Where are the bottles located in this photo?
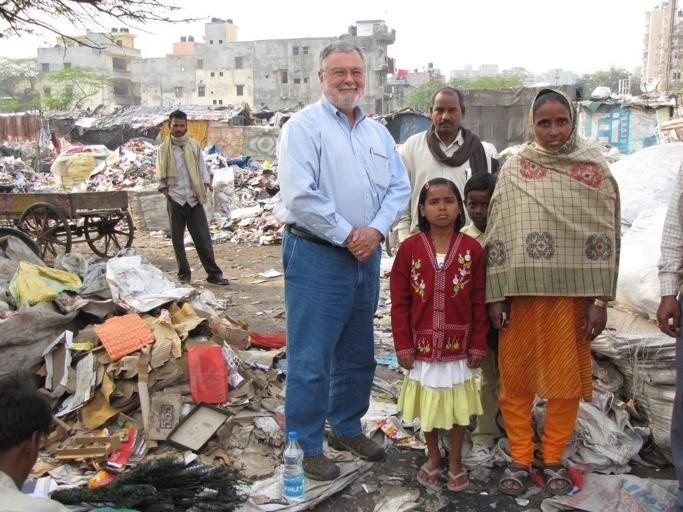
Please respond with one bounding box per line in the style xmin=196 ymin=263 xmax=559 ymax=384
xmin=283 ymin=431 xmax=305 ymax=504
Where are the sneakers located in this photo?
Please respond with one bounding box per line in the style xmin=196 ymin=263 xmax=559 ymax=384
xmin=328 ymin=430 xmax=385 ymax=461
xmin=177 ymin=277 xmax=191 ymax=284
xmin=206 ymin=276 xmax=228 ymax=285
xmin=303 ymin=454 xmax=340 ymax=481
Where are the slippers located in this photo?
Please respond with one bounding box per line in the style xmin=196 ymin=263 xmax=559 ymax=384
xmin=542 ymin=464 xmax=572 ymax=495
xmin=499 ymin=464 xmax=529 ymax=495
xmin=416 ymin=462 xmax=470 ymax=492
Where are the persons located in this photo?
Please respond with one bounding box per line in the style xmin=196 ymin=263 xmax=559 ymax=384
xmin=157 ymin=110 xmax=229 ymax=284
xmin=656 ymin=155 xmax=683 ymax=512
xmin=0 ymin=369 xmax=73 ymax=512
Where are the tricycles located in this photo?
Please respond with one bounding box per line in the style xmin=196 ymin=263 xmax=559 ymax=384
xmin=0 ymin=188 xmax=136 ymax=268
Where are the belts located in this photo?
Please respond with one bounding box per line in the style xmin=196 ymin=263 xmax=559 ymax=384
xmin=290 ymin=224 xmax=339 ymax=248
